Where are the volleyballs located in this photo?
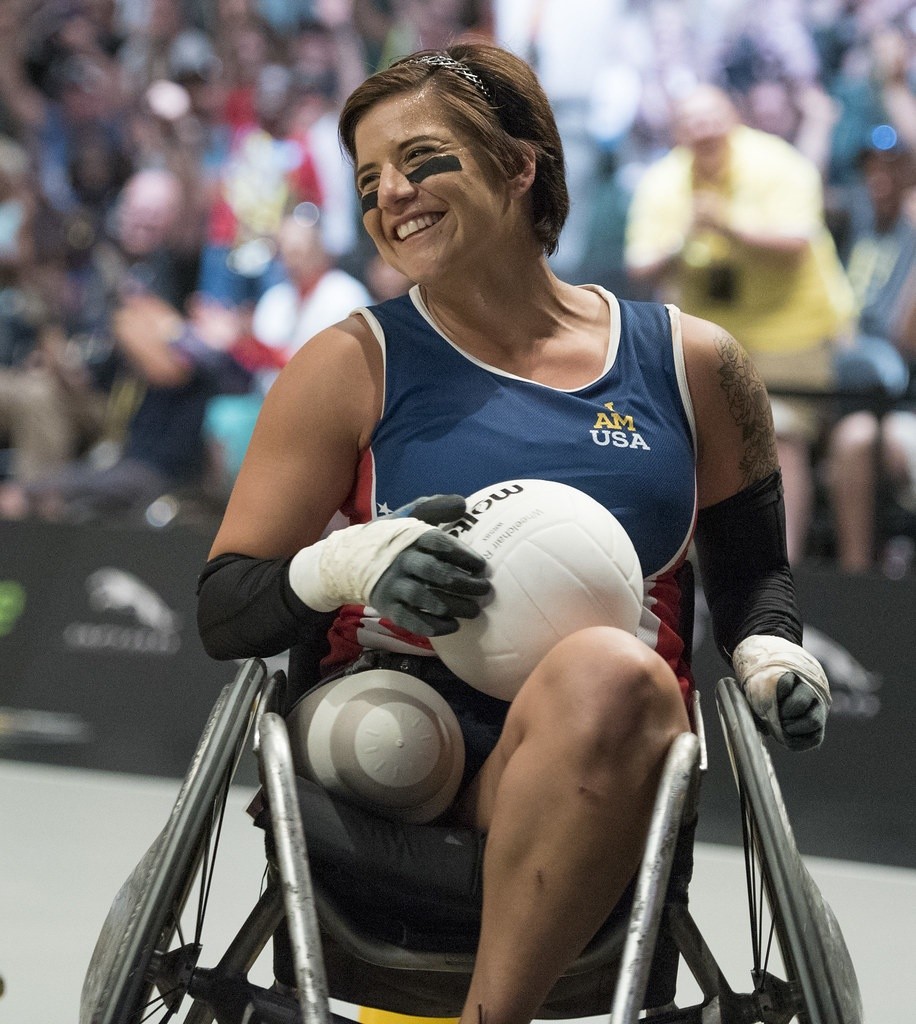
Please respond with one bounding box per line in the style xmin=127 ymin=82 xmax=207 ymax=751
xmin=415 ymin=474 xmax=651 ymax=705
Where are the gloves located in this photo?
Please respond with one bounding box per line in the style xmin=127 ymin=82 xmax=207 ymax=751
xmin=289 ymin=494 xmax=490 ymax=638
xmin=734 ymin=634 xmax=829 ymax=753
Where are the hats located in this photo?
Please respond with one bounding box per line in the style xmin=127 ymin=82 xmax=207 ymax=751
xmin=671 ymin=87 xmax=735 ymax=145
xmin=168 ymin=30 xmax=217 ymax=81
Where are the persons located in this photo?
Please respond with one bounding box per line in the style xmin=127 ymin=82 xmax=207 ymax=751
xmin=196 ymin=45 xmax=827 ymax=1024
xmin=622 ymin=1 xmax=916 ymax=570
xmin=0 ymin=1 xmax=502 ymax=519
